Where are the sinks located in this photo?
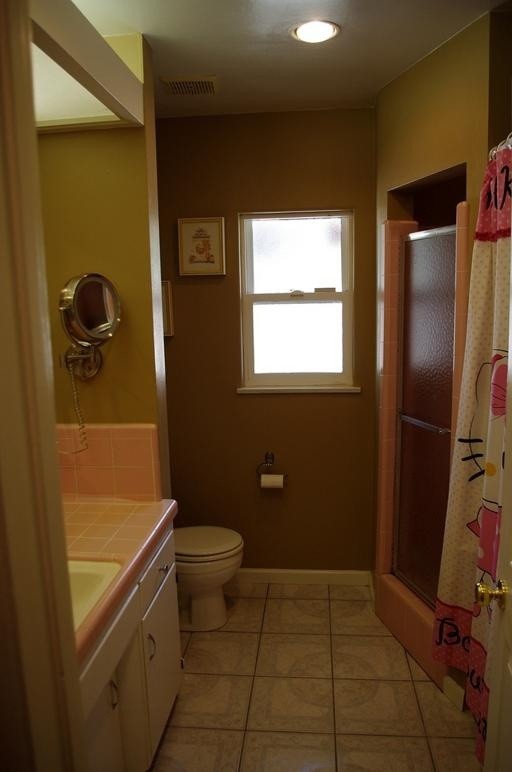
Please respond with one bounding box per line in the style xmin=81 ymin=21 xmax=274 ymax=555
xmin=67 ymin=559 xmax=122 ymax=633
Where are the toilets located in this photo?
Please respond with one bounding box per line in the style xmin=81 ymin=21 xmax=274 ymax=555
xmin=172 ymin=525 xmax=246 ymax=633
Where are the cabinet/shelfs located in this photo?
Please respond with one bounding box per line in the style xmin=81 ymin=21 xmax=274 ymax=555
xmin=72 ymin=516 xmax=185 ymax=772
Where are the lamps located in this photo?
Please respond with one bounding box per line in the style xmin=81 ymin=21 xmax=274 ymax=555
xmin=290 ymin=21 xmax=340 ymax=44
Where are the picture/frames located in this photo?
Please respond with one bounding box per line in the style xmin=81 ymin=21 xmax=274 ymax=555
xmin=177 ymin=217 xmax=226 ymax=277
xmin=161 ymin=280 xmax=175 ymax=337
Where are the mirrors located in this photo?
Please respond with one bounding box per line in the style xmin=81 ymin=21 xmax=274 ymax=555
xmin=58 ymin=273 xmax=124 ymax=381
